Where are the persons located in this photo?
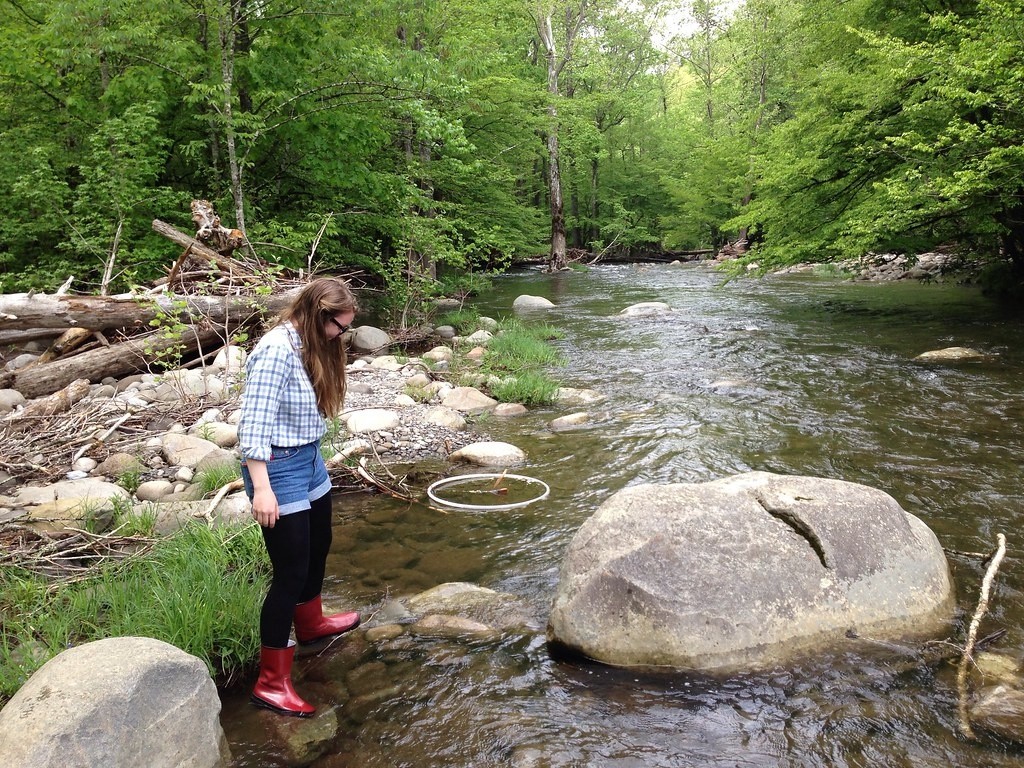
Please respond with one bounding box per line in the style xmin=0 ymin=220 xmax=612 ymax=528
xmin=237 ymin=277 xmax=361 ymax=714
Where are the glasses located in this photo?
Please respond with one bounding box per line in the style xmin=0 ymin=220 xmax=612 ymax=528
xmin=330 ymin=316 xmax=349 ymax=335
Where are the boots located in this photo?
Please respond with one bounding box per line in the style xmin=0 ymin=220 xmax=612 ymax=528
xmin=249 ymin=639 xmax=316 ymax=716
xmin=292 ymin=593 xmax=361 ymax=646
